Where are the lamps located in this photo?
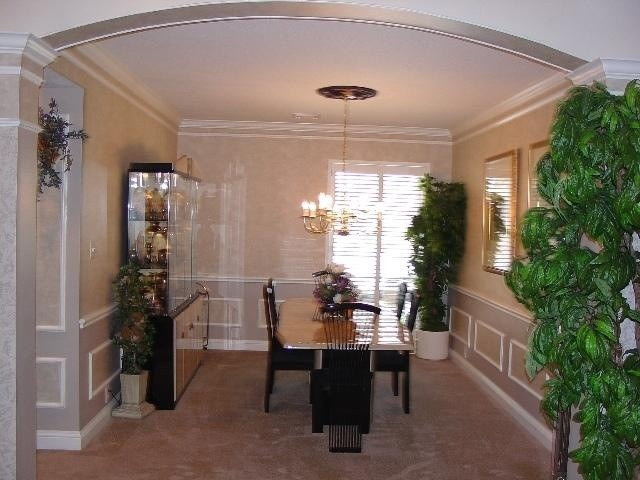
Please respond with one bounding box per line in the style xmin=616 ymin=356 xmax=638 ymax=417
xmin=301 ymin=85 xmax=385 ymax=236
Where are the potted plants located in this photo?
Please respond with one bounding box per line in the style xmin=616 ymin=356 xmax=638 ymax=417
xmin=405 ymin=172 xmax=468 ymax=360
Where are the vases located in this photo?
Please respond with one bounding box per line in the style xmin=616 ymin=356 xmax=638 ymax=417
xmin=113 ymin=370 xmax=155 ymax=418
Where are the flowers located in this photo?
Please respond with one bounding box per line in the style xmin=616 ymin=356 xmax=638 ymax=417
xmin=108 ymin=262 xmax=155 ymax=374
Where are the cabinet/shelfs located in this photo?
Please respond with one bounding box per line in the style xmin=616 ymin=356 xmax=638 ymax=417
xmin=147 ymin=294 xmax=210 ymax=411
xmin=126 ymin=158 xmax=201 ymax=316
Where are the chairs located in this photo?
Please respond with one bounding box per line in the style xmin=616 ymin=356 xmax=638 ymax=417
xmin=262 ymin=270 xmax=421 ymax=434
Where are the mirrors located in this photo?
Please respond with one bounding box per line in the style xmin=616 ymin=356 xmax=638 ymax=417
xmin=481 ymin=148 xmax=519 ymax=276
xmin=527 ymin=139 xmax=552 ymax=208
